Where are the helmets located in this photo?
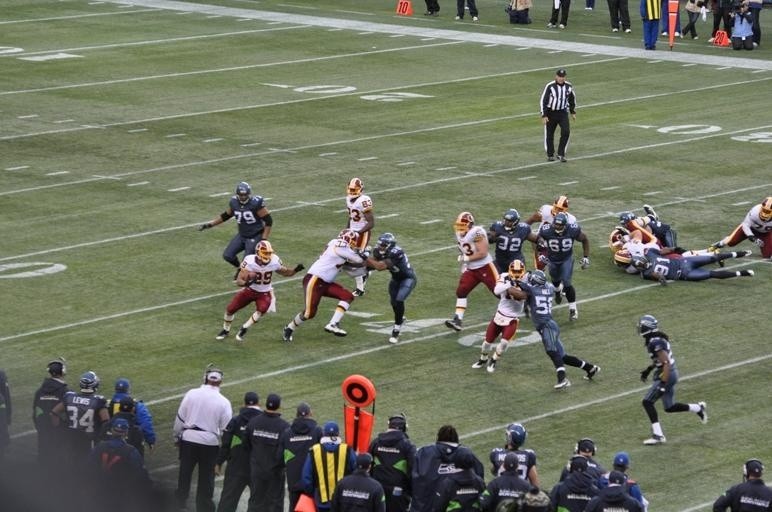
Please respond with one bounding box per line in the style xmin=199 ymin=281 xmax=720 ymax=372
xmin=528 ymin=270 xmax=546 ymax=286
xmin=613 ymin=250 xmax=633 ymax=268
xmin=375 ymin=232 xmax=396 ymax=257
xmin=503 ymin=208 xmax=520 ymax=232
xmin=255 ymin=240 xmax=273 ymax=265
xmin=608 ymin=229 xmax=624 ymax=254
xmin=80 ymin=371 xmax=101 ymax=394
xmin=506 ymin=422 xmax=527 ymax=449
xmin=636 ymin=314 xmax=658 ymax=337
xmin=550 ymin=195 xmax=570 ymax=217
xmin=552 ymin=214 xmax=568 ymax=235
xmin=760 ymin=197 xmax=771 ymax=221
xmin=619 ymin=212 xmax=636 ymax=227
xmin=346 ymin=177 xmax=364 ymax=198
xmin=454 ymin=211 xmax=475 ymax=237
xmin=508 ymin=259 xmax=525 ymax=280
xmin=338 ymin=229 xmax=358 ymax=251
xmin=234 ymin=181 xmax=252 ymax=206
xmin=47 ymin=358 xmax=66 ymax=378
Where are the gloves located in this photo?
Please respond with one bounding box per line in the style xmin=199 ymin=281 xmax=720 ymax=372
xmin=195 ymin=223 xmax=212 ymax=231
xmin=363 ymin=244 xmax=372 ymax=254
xmin=660 ymin=277 xmax=667 ymax=287
xmin=456 ymin=255 xmax=470 ymax=264
xmin=355 ymin=252 xmax=368 ymax=262
xmin=580 ymin=255 xmax=590 ymax=269
xmin=753 ymin=238 xmax=765 ymax=248
xmin=675 ymin=247 xmax=687 ymax=255
xmin=536 ymin=243 xmax=545 ymax=252
xmin=538 ymin=255 xmax=550 ymax=266
xmin=295 ymin=263 xmax=305 ymax=273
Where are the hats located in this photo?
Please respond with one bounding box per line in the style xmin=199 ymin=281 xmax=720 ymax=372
xmin=386 ymin=412 xmax=407 ymax=425
xmin=504 ymin=452 xmax=518 ymax=472
xmin=203 ymin=365 xmax=223 ymax=385
xmin=743 ymin=459 xmax=763 ymax=478
xmin=567 ymin=439 xmax=628 ymax=485
xmin=357 ymin=453 xmax=377 ymax=471
xmin=556 ymin=69 xmax=567 ymax=76
xmin=244 ymin=391 xmax=339 ymax=439
xmin=519 ymin=487 xmax=553 ymax=510
xmin=110 ymin=377 xmax=136 ymax=433
xmin=453 ymin=448 xmax=477 ymax=470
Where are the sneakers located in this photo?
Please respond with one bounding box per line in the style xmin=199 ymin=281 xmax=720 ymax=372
xmin=546 ymin=155 xmax=567 ymax=163
xmin=472 ymin=360 xmax=487 ymax=368
xmin=644 ymin=204 xmax=659 ymax=222
xmin=554 ymin=379 xmax=571 ymax=389
xmin=583 ymin=365 xmax=601 ymax=380
xmin=235 ymin=324 xmax=246 ymax=341
xmin=554 ymin=290 xmax=563 ymax=304
xmin=706 ymin=240 xmax=754 ymax=278
xmin=395 ymin=316 xmax=406 ymax=330
xmin=281 ymin=323 xmax=294 ymax=344
xmin=643 ymin=434 xmax=667 ymax=446
xmin=698 ymin=402 xmax=708 ymax=426
xmin=443 ymin=316 xmax=462 ymax=331
xmin=568 ymin=307 xmax=578 ymax=322
xmin=389 ymin=329 xmax=400 ymax=344
xmin=487 ymin=360 xmax=497 ymax=373
xmin=323 ymin=322 xmax=348 ymax=338
xmin=353 ymin=289 xmax=365 ymax=298
xmin=216 ymin=329 xmax=230 ymax=340
xmin=423 ymin=6 xmax=759 ymax=50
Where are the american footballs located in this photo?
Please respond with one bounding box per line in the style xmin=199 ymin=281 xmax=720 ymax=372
xmin=248 ymin=272 xmax=256 ymax=280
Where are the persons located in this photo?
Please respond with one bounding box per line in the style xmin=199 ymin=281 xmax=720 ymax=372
xmin=200 ymin=179 xmax=417 ymax=344
xmin=445 ymin=195 xmax=600 ymax=390
xmin=423 ymin=0 xmax=764 ymax=50
xmin=712 ymin=458 xmax=771 ymax=512
xmin=609 ymin=177 xmax=771 ymax=282
xmin=539 ymin=69 xmax=577 ymax=162
xmin=636 ymin=314 xmax=707 ymax=445
xmin=1 ymin=360 xmax=649 ymax=511
